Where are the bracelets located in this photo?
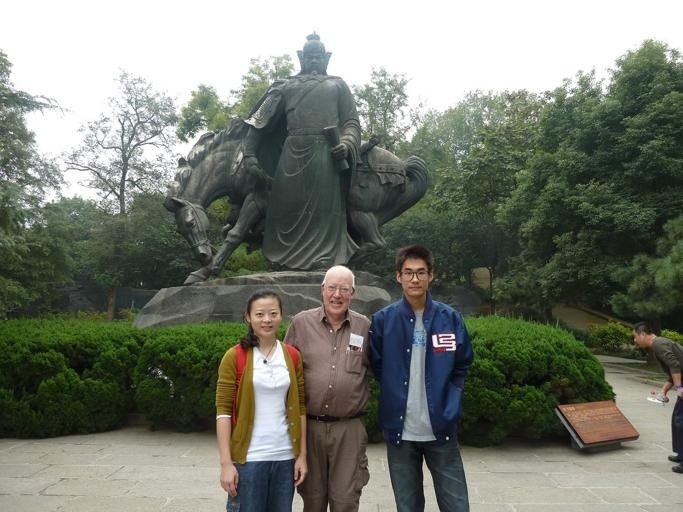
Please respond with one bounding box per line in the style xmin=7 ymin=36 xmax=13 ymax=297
xmin=673 ymin=384 xmax=681 ymax=389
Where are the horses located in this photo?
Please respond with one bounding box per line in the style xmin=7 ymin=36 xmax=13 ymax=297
xmin=157 ymin=113 xmax=431 ymax=286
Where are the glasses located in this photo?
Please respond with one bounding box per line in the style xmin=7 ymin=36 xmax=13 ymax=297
xmin=398 ymin=269 xmax=430 ymax=282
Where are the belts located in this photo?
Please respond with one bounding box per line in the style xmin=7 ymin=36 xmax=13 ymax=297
xmin=302 ymin=410 xmax=370 ymax=424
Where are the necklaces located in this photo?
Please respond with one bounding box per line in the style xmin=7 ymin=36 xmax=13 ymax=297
xmin=263 ymin=340 xmax=276 ymax=364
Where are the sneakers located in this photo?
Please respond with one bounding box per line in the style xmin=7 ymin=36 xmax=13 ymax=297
xmin=668 ymin=453 xmax=683 ymax=473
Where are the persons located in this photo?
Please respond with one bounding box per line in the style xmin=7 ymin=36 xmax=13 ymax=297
xmin=240 ymin=28 xmax=364 ymax=272
xmin=282 ymin=265 xmax=373 ymax=512
xmin=214 ymin=291 xmax=308 ymax=512
xmin=367 ymin=245 xmax=475 ymax=512
xmin=632 ymin=321 xmax=683 ymax=473
